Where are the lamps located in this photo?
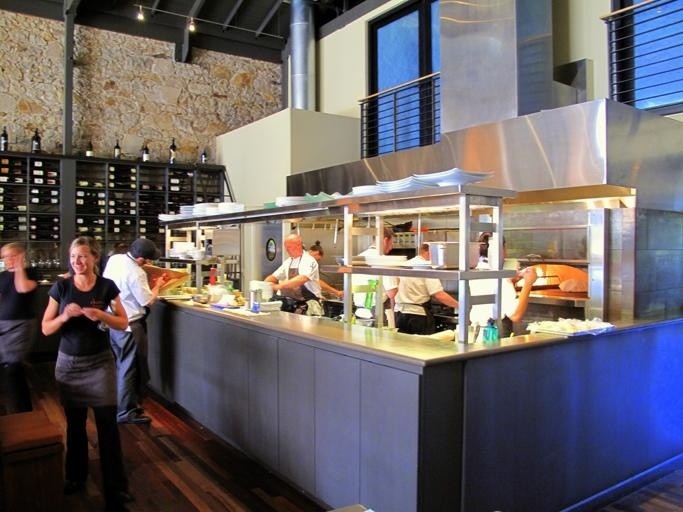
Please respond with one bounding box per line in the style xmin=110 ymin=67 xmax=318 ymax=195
xmin=136 ymin=3 xmax=145 ymax=22
xmin=188 ymin=16 xmax=198 ymax=33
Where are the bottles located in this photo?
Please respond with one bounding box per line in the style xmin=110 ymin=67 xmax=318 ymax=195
xmin=0 ymin=126 xmax=221 ymax=241
xmin=482 ymin=318 xmax=499 ymax=348
xmin=249 ymin=291 xmax=262 ymax=313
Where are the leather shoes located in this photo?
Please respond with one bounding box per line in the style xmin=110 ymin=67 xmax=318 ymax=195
xmin=118 ymin=408 xmax=152 ymax=424
xmin=64 ymin=480 xmax=132 ymax=504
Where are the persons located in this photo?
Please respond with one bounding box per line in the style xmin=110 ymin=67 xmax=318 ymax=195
xmin=466 ymin=233 xmax=537 ymax=337
xmin=264 ymin=234 xmax=343 ymax=315
xmin=101 ymin=239 xmax=166 ymax=423
xmin=41 ymin=236 xmax=135 ymax=503
xmin=349 ymin=229 xmax=397 ymax=327
xmin=0 ymin=242 xmax=47 ymax=413
xmin=395 ymin=242 xmax=458 ymax=334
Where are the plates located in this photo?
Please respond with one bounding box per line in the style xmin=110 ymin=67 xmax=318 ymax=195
xmin=275 ymin=196 xmax=305 ymax=207
xmin=352 ymin=167 xmax=495 ymax=196
xmin=158 ymin=201 xmax=244 ymax=222
xmin=366 ymin=255 xmax=407 ymax=270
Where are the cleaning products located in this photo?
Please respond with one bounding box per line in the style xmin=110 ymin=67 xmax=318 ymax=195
xmin=484 ymin=319 xmax=499 ymax=346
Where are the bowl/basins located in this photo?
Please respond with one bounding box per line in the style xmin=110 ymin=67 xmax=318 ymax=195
xmin=423 ymin=240 xmax=486 ymax=270
xmin=192 ymin=294 xmax=211 ymax=305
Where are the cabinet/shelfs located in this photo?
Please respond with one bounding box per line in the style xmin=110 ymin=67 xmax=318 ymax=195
xmin=0 ymin=151 xmax=225 ymax=282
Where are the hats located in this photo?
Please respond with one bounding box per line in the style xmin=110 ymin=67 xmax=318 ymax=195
xmin=130 ymin=239 xmax=162 ymax=260
